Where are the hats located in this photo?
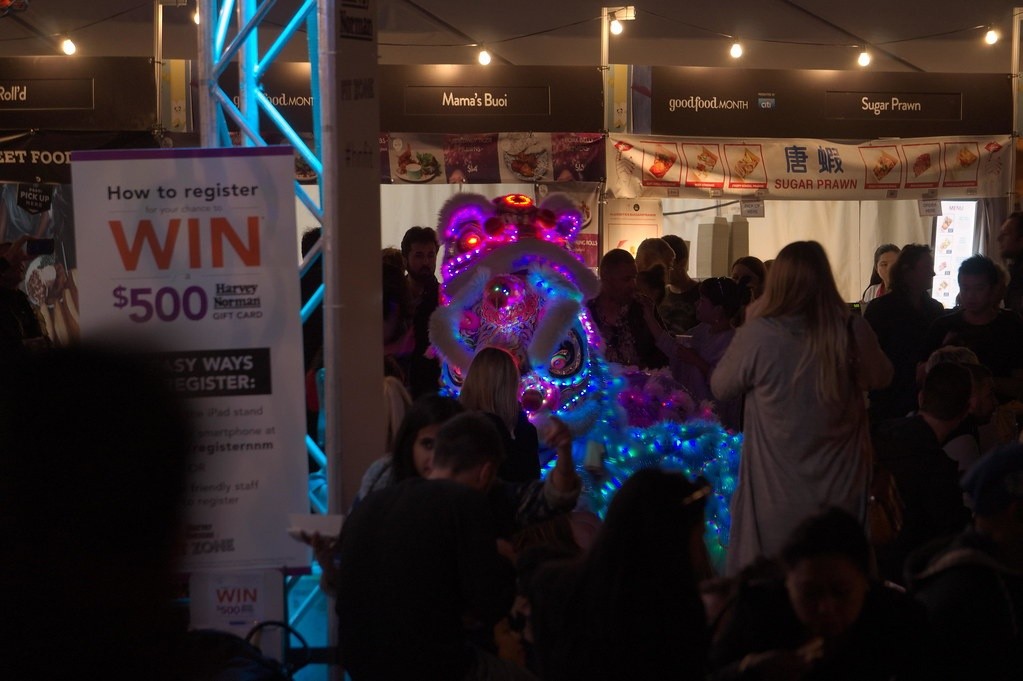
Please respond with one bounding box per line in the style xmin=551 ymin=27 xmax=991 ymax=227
xmin=960 ymin=442 xmax=1023 ymax=516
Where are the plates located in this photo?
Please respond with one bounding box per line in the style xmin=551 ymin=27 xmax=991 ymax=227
xmin=281 ymin=139 xmax=318 ymax=181
xmin=503 ymin=147 xmax=550 ymax=182
xmin=395 ymin=166 xmax=436 ymax=182
xmin=23 ymin=255 xmax=67 ymax=308
xmin=577 ymin=202 xmax=592 ymax=228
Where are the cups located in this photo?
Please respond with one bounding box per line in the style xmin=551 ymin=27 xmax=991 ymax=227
xmin=405 ymin=164 xmax=422 ymax=178
xmin=677 ymin=334 xmax=693 ymax=350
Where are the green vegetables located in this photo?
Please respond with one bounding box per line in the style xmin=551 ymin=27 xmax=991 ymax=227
xmin=417 ymin=153 xmax=442 ymax=175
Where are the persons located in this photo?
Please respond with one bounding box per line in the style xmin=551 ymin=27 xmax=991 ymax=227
xmin=710 ymin=241 xmax=893 ymax=586
xmin=300 ymin=212 xmax=1023 ymax=680
xmin=0 ymin=219 xmax=291 ymax=681
